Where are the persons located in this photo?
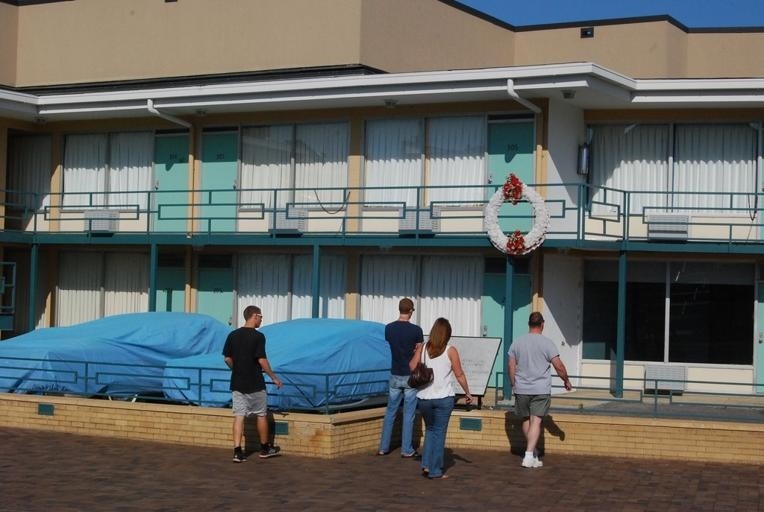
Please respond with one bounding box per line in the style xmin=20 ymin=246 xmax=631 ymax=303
xmin=377 ymin=298 xmax=424 ymax=458
xmin=408 ymin=318 xmax=472 ymax=480
xmin=222 ymin=306 xmax=281 ymax=462
xmin=508 ymin=313 xmax=571 ymax=469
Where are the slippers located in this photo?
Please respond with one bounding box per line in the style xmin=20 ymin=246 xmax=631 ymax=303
xmin=402 ymin=451 xmax=422 ymax=458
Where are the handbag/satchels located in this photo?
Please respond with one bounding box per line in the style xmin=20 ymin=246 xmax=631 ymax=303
xmin=408 ymin=362 xmax=433 ymax=388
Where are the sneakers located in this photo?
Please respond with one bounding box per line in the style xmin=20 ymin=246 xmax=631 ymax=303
xmin=233 ymin=451 xmax=248 ymax=462
xmin=522 ymin=448 xmax=543 ymax=468
xmin=259 ymin=443 xmax=280 ymax=458
